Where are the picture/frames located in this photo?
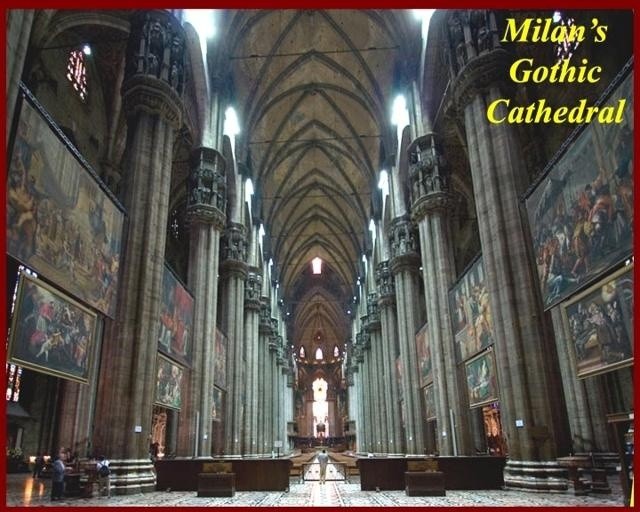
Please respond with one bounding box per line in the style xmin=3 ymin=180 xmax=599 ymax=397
xmin=154 ymin=352 xmax=189 ymax=413
xmin=465 ymin=350 xmax=499 ymax=409
xmin=6 ymin=269 xmax=99 ymax=385
xmin=559 ymin=261 xmax=634 ymax=381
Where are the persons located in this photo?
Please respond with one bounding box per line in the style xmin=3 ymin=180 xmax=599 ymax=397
xmin=317 ymin=449 xmax=329 ymax=485
xmin=95 ymin=455 xmax=111 ymax=499
xmin=567 ymin=434 xmax=600 ymax=484
xmin=31 ymin=443 xmax=75 ymax=501
xmin=415 ymin=123 xmax=633 ymax=455
xmin=6 ymin=128 xmax=228 ymax=420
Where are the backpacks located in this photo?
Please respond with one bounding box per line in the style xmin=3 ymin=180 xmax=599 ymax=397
xmin=99 ymin=460 xmax=111 ymax=478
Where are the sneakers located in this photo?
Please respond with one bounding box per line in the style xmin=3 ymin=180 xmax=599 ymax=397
xmin=50 ymin=497 xmax=64 ymax=502
